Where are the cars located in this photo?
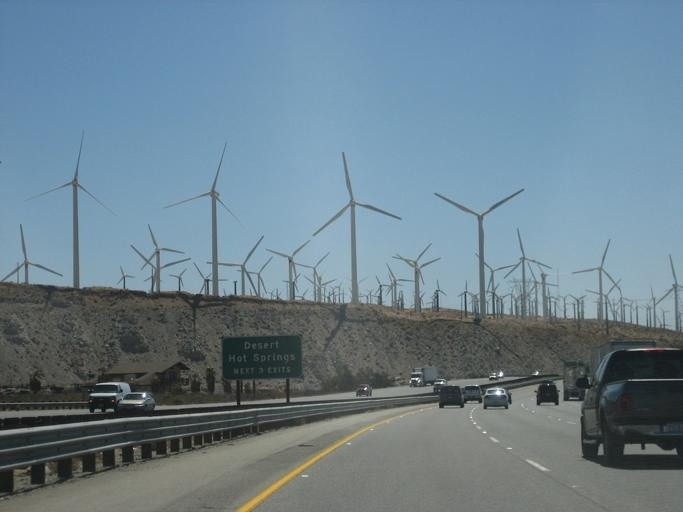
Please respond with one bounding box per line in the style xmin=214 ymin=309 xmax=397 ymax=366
xmin=355 ymin=383 xmax=373 ymax=397
xmin=430 ymin=369 xmax=563 ymax=410
xmin=115 ymin=390 xmax=157 ymax=414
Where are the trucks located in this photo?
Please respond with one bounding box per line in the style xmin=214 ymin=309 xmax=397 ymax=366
xmin=595 ymin=339 xmax=659 ymax=363
xmin=561 ymin=359 xmax=587 ymax=404
xmin=86 ymin=380 xmax=132 ymax=416
xmin=408 ymin=365 xmax=438 ymax=388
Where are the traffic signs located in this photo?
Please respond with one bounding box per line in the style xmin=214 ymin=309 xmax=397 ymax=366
xmin=220 ymin=335 xmax=304 ymax=381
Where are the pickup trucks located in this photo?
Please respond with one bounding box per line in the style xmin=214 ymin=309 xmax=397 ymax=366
xmin=569 ymin=344 xmax=682 ymax=465
